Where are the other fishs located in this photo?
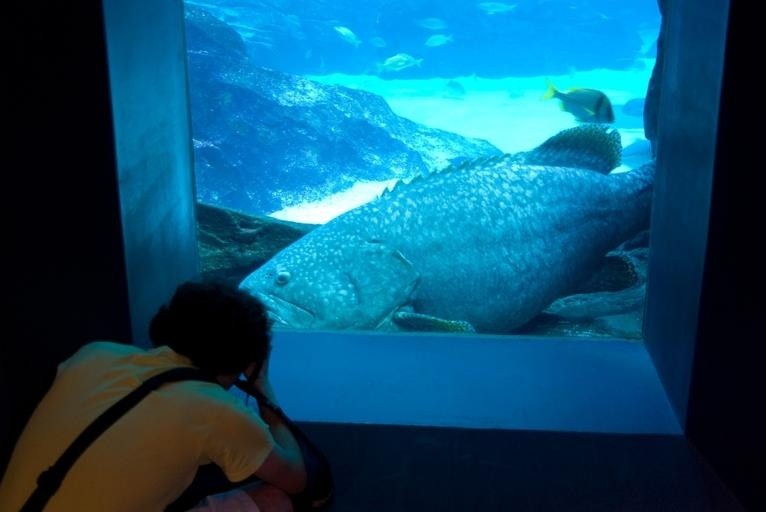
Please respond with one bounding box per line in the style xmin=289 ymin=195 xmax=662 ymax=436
xmin=238 ymin=125 xmax=656 ymax=334
xmin=329 ymin=1 xmax=654 ymax=123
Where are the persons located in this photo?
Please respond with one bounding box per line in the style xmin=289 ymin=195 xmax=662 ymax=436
xmin=0 ymin=279 xmax=308 ymax=511
